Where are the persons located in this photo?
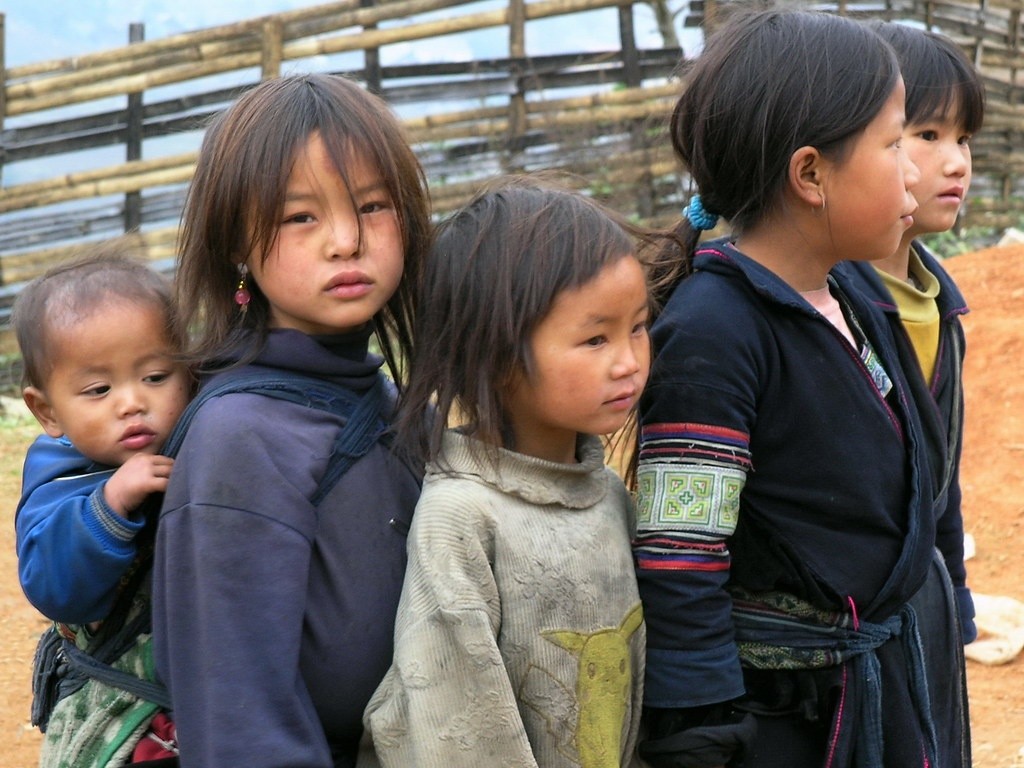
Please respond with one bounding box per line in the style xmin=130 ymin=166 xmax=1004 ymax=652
xmin=13 ymin=247 xmax=191 ymax=768
xmin=152 ymin=70 xmax=440 ymax=768
xmin=354 ymin=187 xmax=648 ymax=767
xmin=857 ymin=13 xmax=987 ymax=768
xmin=608 ymin=7 xmax=974 ymax=768
